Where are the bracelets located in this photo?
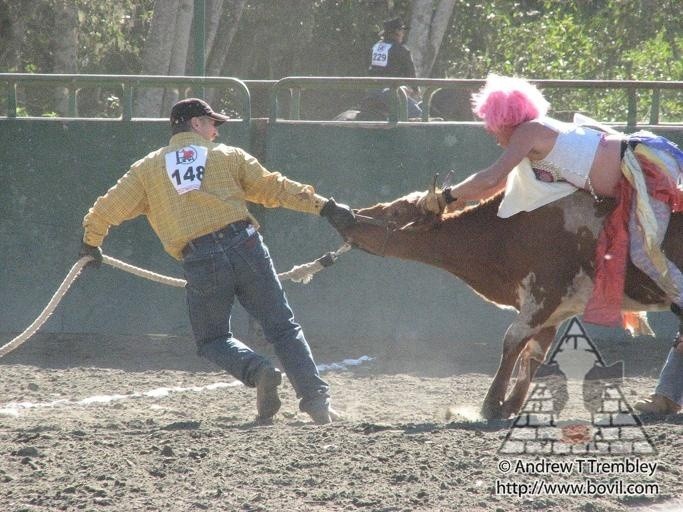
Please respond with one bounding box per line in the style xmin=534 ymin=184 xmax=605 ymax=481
xmin=439 ymin=187 xmax=458 ymax=204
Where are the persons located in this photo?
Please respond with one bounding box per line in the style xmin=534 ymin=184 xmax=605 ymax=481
xmin=361 ymin=16 xmax=423 ymax=121
xmin=631 ymin=332 xmax=681 ymax=417
xmin=413 ymin=72 xmax=682 ymax=357
xmin=77 ymin=97 xmax=362 ymax=426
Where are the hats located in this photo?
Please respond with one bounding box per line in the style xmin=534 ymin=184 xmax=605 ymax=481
xmin=170 ymin=97 xmax=230 ymax=126
xmin=384 ymin=15 xmax=407 ymax=31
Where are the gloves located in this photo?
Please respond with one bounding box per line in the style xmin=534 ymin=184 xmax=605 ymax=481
xmin=320 ymin=197 xmax=357 ymax=226
xmin=79 ymin=244 xmax=102 ymax=268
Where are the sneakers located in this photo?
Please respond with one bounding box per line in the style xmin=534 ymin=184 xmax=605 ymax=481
xmin=309 ymin=406 xmax=331 ymax=423
xmin=634 ymin=394 xmax=681 ymax=415
xmin=256 ymin=367 xmax=282 ymax=417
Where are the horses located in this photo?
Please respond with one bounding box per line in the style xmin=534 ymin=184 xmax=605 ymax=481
xmin=329 ymin=87 xmax=478 ymax=121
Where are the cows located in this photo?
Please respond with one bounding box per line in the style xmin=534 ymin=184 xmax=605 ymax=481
xmin=344 ymin=170 xmax=683 ymax=421
